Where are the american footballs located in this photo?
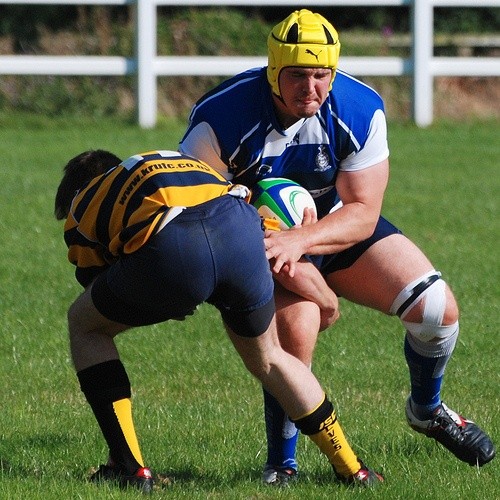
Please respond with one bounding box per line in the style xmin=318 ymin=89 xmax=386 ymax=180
xmin=252 ymin=178 xmax=317 ymax=231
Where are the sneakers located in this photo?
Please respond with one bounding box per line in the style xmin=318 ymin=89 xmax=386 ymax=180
xmin=90 ymin=465 xmax=153 ymax=494
xmin=263 ymin=463 xmax=297 ymax=487
xmin=405 ymin=396 xmax=494 ymax=466
xmin=332 ymin=458 xmax=384 ymax=489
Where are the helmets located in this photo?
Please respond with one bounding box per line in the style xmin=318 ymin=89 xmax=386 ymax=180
xmin=267 ymin=9 xmax=340 ymax=98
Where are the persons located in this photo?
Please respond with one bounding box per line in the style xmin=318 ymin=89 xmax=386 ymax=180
xmin=179 ymin=9 xmax=496 ymax=488
xmin=54 ymin=149 xmax=386 ymax=492
